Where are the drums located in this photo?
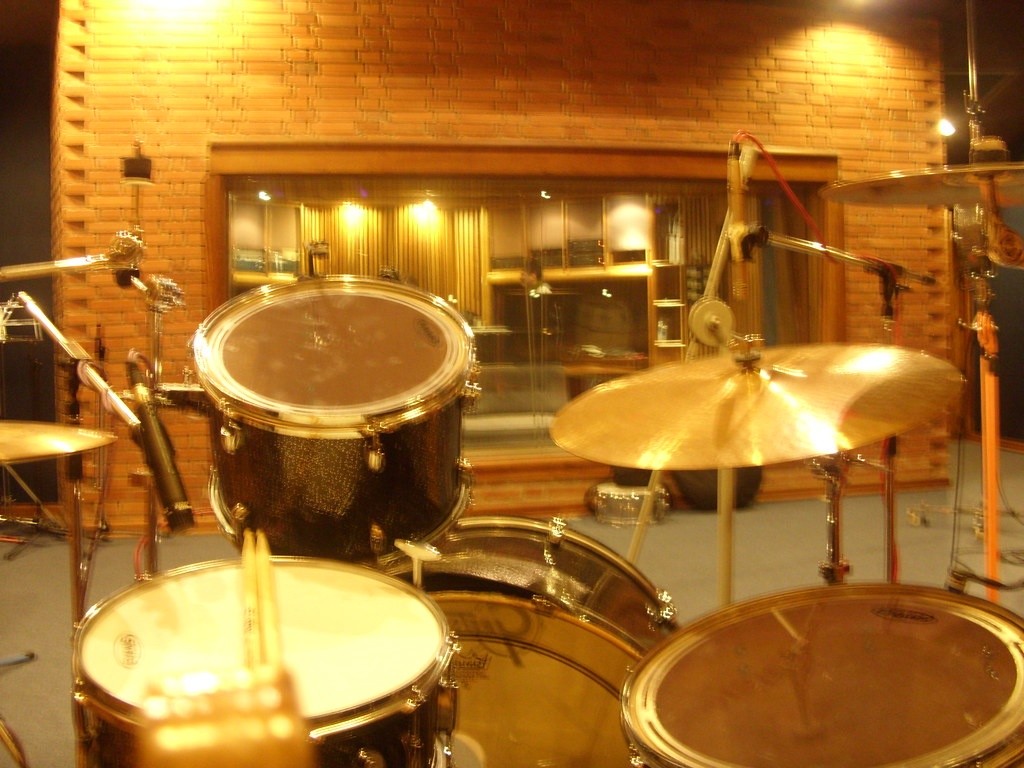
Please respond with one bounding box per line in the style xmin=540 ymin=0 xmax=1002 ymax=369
xmin=366 ymin=513 xmax=679 ymax=768
xmin=69 ymin=554 xmax=461 ymax=768
xmin=593 ymin=482 xmax=669 ymax=525
xmin=616 ymin=582 xmax=1024 ymax=768
xmin=184 ymin=273 xmax=477 ymax=563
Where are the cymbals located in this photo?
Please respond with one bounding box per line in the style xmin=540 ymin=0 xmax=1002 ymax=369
xmin=0 ymin=418 xmax=118 ymax=467
xmin=820 ymin=161 xmax=1024 ymax=210
xmin=547 ymin=342 xmax=966 ymax=473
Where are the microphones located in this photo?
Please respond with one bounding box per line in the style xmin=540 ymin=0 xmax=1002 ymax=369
xmin=727 ymin=141 xmax=756 ymax=266
xmin=123 ymin=361 xmax=196 ymax=536
